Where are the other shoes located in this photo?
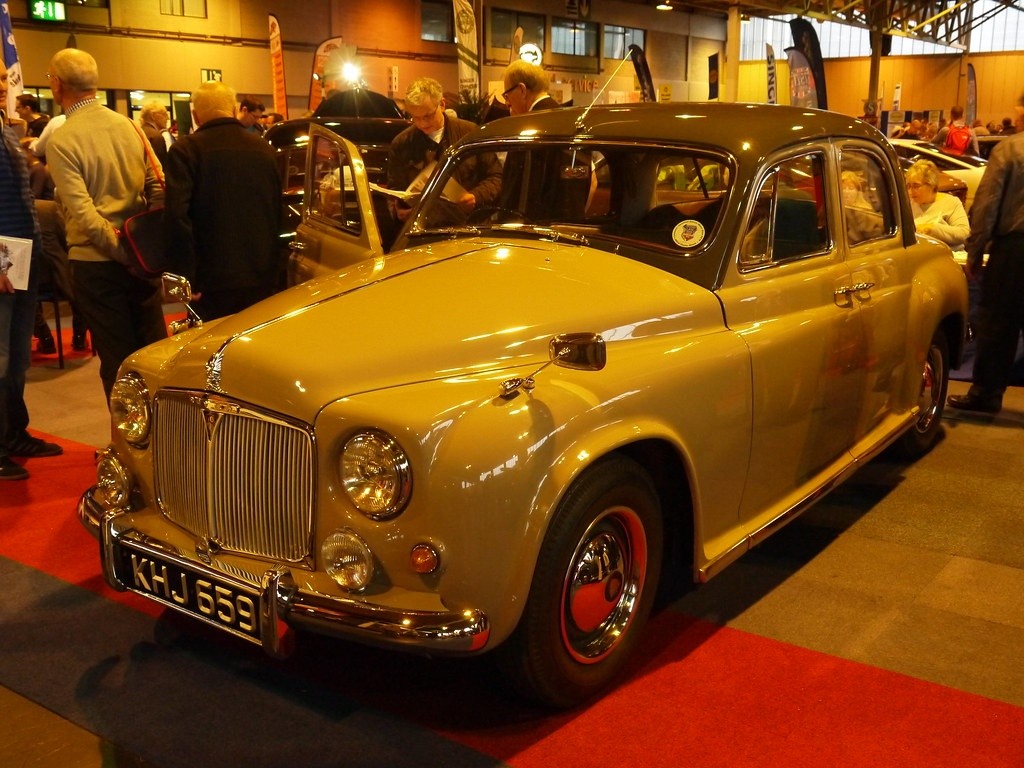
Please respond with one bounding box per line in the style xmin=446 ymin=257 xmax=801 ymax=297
xmin=36 ymin=335 xmax=54 ymax=354
xmin=72 ymin=334 xmax=86 ymax=349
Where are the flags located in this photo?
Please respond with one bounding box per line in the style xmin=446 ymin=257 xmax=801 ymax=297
xmin=0 ymin=0 xmax=25 ymax=120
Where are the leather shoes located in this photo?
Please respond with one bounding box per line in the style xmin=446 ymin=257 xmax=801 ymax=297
xmin=947 ymin=395 xmax=1000 ymax=413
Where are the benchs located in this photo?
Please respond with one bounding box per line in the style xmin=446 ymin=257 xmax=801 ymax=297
xmin=746 ymin=235 xmax=823 ymax=261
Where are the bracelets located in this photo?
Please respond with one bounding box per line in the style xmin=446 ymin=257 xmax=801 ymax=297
xmin=28 ymin=162 xmax=41 ymax=166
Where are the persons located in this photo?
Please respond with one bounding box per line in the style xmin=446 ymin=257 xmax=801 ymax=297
xmin=15 ymin=93 xmax=55 ymax=201
xmin=386 ymin=75 xmax=504 ymax=225
xmin=44 ymin=48 xmax=169 ymax=461
xmin=139 ymin=101 xmax=168 ymax=162
xmin=497 ymin=57 xmax=592 ymax=224
xmin=0 ymin=57 xmax=63 ymax=480
xmin=237 ymin=97 xmax=266 ymax=128
xmin=266 ymin=113 xmax=284 ymax=131
xmin=18 ymin=115 xmax=66 ymax=157
xmin=841 ymin=171 xmax=873 ymax=210
xmin=890 ymin=92 xmax=1024 ymax=411
xmin=33 ymin=200 xmax=90 ymax=353
xmin=166 ymin=80 xmax=284 ymax=329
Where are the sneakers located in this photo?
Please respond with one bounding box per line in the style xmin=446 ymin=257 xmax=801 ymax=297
xmin=0 ymin=457 xmax=28 ymax=478
xmin=7 ymin=434 xmax=62 ymax=456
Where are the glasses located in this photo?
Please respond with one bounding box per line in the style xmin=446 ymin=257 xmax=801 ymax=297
xmin=407 ymin=102 xmax=440 ymax=123
xmin=502 ymin=82 xmax=528 ymax=102
xmin=45 ymin=72 xmax=62 ymax=81
xmin=905 ymin=179 xmax=929 ymax=190
xmin=247 ymin=109 xmax=260 ymax=120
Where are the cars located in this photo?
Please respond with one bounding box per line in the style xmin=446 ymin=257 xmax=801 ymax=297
xmin=260 ymin=113 xmax=407 ymax=225
xmin=585 ymin=135 xmax=989 ymax=223
xmin=75 ymin=103 xmax=970 ymax=717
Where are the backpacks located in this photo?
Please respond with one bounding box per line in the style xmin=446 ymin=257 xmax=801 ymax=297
xmin=946 ymin=123 xmax=972 ymax=153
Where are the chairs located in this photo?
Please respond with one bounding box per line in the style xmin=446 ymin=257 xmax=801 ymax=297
xmin=36 ymin=257 xmax=98 ymax=370
